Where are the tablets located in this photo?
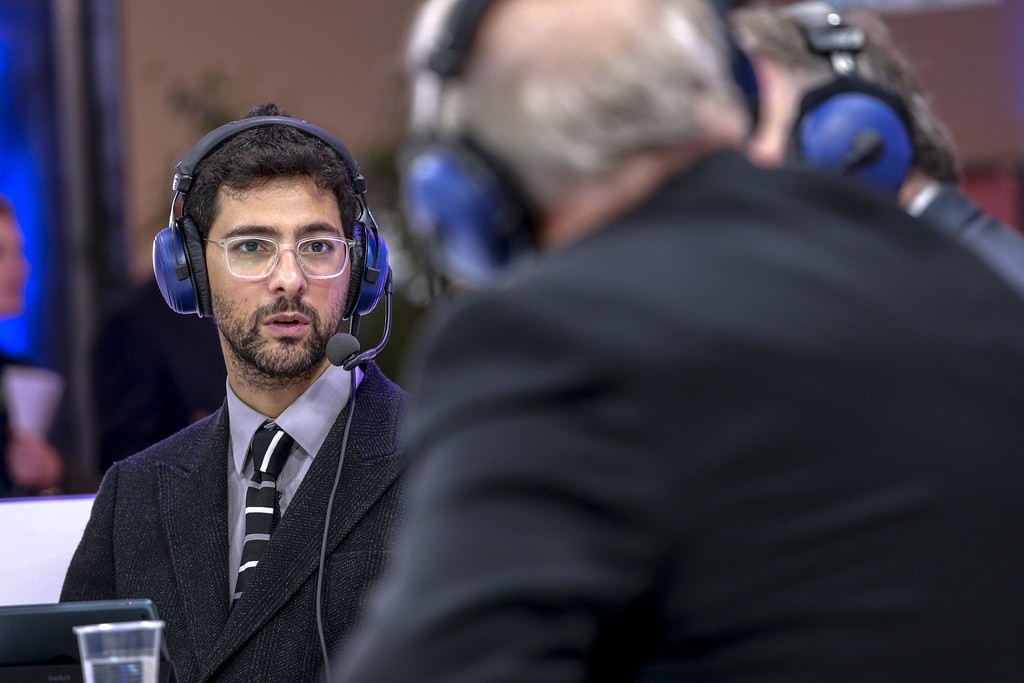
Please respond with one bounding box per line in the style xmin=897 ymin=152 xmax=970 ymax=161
xmin=0 ymin=600 xmax=180 ymax=683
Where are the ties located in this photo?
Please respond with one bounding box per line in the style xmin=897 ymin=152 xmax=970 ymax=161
xmin=230 ymin=424 xmax=299 ymax=617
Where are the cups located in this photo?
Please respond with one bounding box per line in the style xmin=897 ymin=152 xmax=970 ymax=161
xmin=72 ymin=619 xmax=166 ymax=682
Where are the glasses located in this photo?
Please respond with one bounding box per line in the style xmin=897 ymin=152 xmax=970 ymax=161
xmin=203 ymin=234 xmax=357 ymax=282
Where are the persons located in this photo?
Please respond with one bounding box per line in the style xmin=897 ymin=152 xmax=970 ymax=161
xmin=326 ymin=1 xmax=1024 ymax=683
xmin=0 ymin=196 xmax=78 ymax=500
xmin=58 ymin=104 xmax=422 ymax=683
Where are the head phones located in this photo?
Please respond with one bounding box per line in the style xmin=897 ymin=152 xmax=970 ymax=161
xmin=151 ymin=116 xmax=393 ymax=320
xmin=783 ymin=3 xmax=920 ymax=202
xmin=394 ymin=0 xmax=763 ymax=286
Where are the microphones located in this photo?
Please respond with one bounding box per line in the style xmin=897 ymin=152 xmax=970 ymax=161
xmin=326 ymin=293 xmax=393 ymax=371
